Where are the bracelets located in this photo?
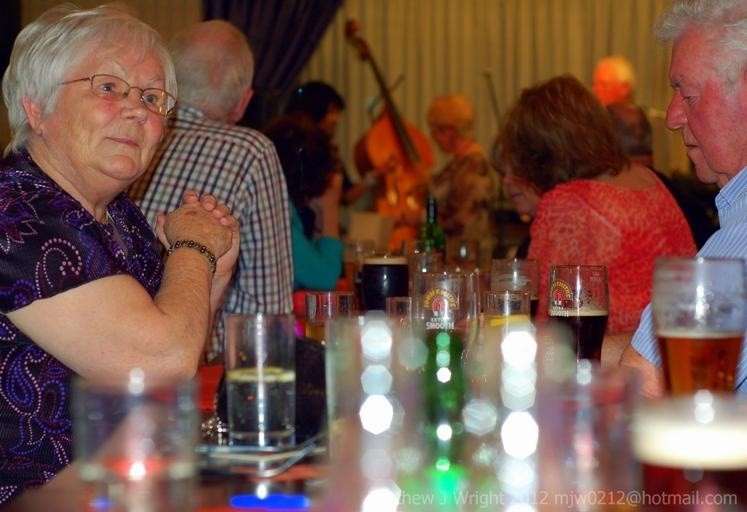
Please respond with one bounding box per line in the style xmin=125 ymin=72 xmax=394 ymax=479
xmin=161 ymin=238 xmax=217 ymax=274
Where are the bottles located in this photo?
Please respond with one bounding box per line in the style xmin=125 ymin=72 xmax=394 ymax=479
xmin=416 ymin=195 xmax=448 ymax=267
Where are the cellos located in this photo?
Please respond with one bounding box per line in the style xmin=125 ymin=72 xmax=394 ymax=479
xmin=340 ymin=18 xmax=437 ymax=250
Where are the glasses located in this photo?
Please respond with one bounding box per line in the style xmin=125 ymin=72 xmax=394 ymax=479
xmin=58 ymin=74 xmax=178 ymax=118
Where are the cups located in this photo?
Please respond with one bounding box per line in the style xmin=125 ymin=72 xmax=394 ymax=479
xmin=67 ymin=232 xmax=747 ymax=511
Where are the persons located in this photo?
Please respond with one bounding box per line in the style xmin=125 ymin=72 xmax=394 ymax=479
xmin=407 ymin=89 xmax=502 ymax=268
xmin=595 ymin=0 xmax=747 ymax=405
xmin=491 ymin=72 xmax=701 ymax=376
xmin=252 ymin=110 xmax=346 ymax=296
xmin=282 ymin=78 xmax=400 ymax=239
xmin=485 ymin=150 xmax=539 ymax=316
xmin=122 ymin=17 xmax=327 ymax=452
xmin=606 ymin=97 xmax=716 ymax=252
xmin=590 ymin=52 xmax=640 ymax=110
xmin=0 ymin=0 xmax=243 ymax=511
